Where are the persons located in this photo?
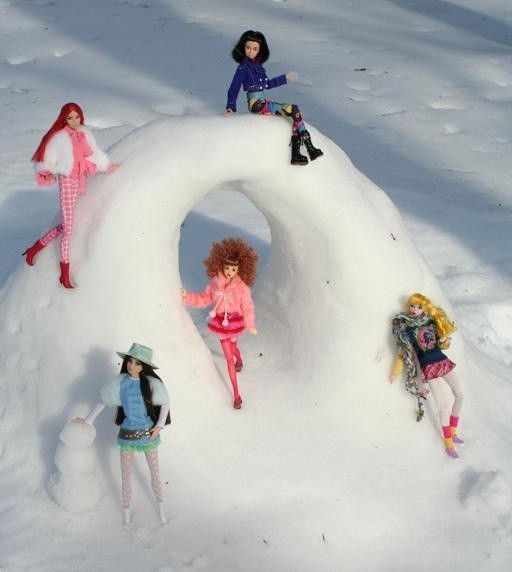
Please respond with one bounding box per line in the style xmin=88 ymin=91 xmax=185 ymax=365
xmin=21 ymin=103 xmax=121 ymax=288
xmin=389 ymin=294 xmax=466 ymax=460
xmin=225 ymin=27 xmax=322 ymax=165
xmin=180 ymin=237 xmax=258 ymax=410
xmin=73 ymin=343 xmax=172 ymax=528
